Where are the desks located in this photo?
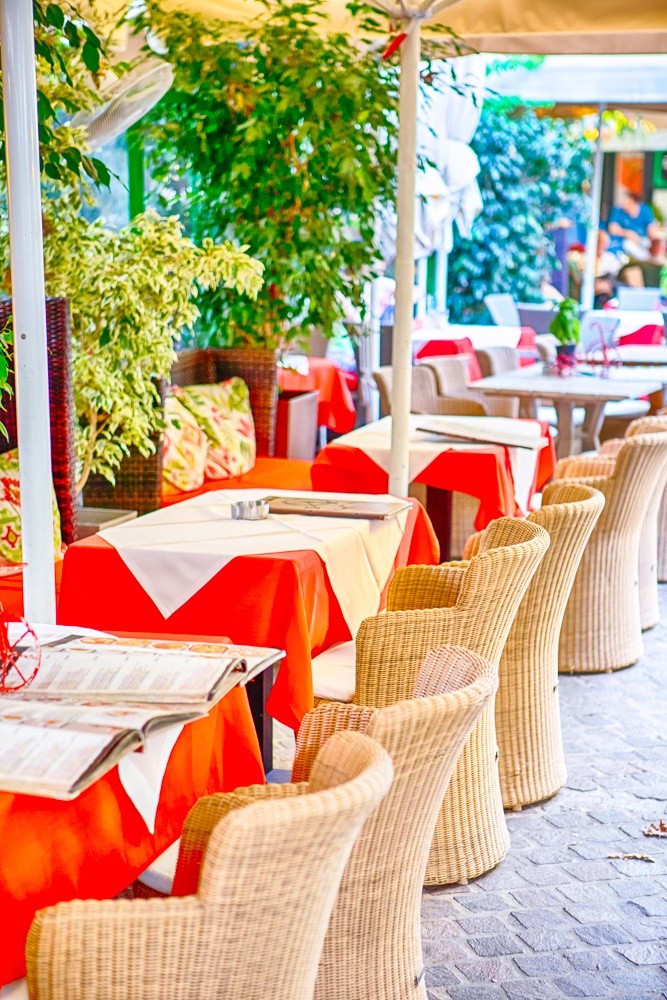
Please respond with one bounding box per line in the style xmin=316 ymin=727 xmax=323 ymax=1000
xmin=63 ymin=487 xmax=442 ymax=774
xmin=467 ymin=345 xmax=665 ymax=453
xmin=0 ymin=630 xmax=265 ymax=986
xmin=311 ymin=412 xmax=557 ymax=561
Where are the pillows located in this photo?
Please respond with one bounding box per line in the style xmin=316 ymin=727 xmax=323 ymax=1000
xmin=181 ymin=378 xmax=255 ymax=480
xmin=161 ymin=394 xmax=206 ymax=494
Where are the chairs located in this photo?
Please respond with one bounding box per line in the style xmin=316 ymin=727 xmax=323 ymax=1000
xmin=24 ymin=286 xmax=667 ymax=1000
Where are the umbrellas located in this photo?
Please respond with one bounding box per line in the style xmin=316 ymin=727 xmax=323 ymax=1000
xmin=0 ymin=0 xmax=667 ymax=625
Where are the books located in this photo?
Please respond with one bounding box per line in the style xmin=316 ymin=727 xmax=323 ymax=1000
xmin=258 ymin=496 xmax=413 ymax=520
xmin=415 ymin=419 xmax=548 ymax=451
xmin=0 ymin=632 xmax=287 ymax=801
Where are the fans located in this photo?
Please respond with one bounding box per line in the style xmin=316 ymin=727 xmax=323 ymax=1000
xmin=70 ymin=59 xmax=178 ymax=151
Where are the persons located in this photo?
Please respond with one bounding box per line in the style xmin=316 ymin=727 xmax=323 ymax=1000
xmin=571 ymin=190 xmax=667 ymax=300
